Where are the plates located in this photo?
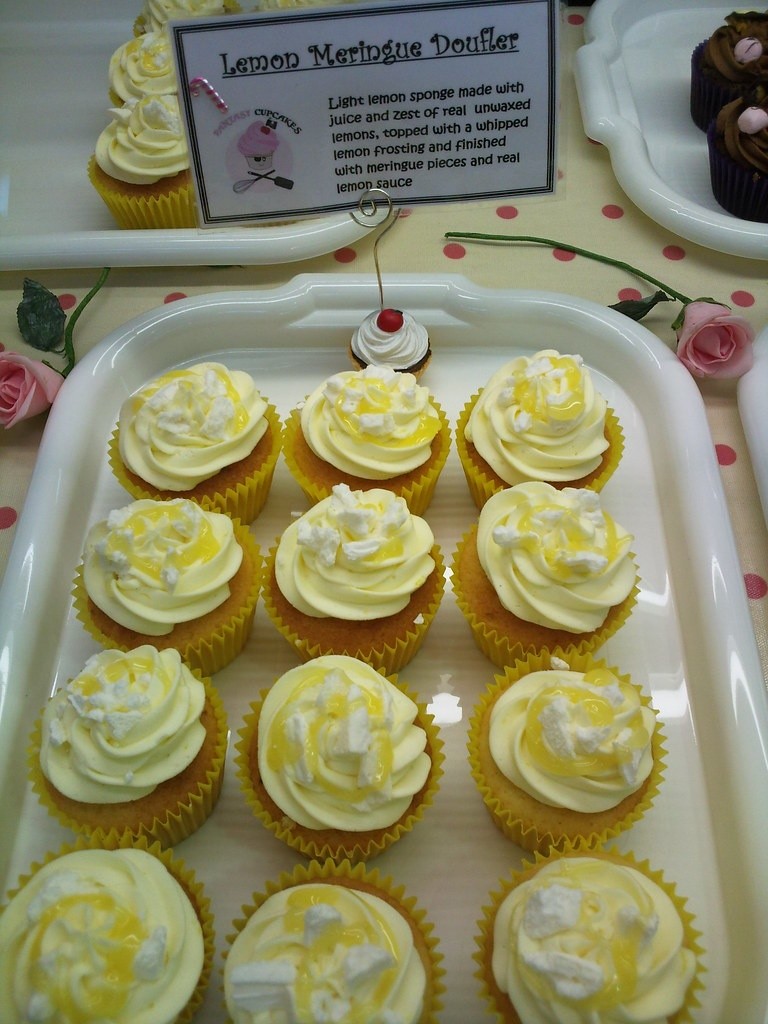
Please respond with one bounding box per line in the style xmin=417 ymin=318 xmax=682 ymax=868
xmin=578 ymin=1 xmax=768 ymax=263
xmin=1 ymin=1 xmax=388 ymax=274
xmin=1 ymin=267 xmax=768 ymax=1024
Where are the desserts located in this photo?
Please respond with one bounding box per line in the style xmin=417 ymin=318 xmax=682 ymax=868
xmin=0 ymin=0 xmax=766 ymax=1024
